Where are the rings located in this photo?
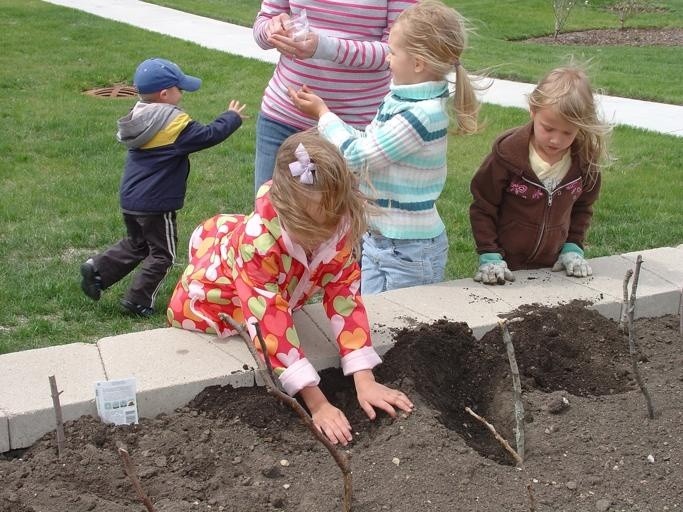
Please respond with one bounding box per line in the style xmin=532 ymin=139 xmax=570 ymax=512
xmin=292 ymin=49 xmax=298 ymax=58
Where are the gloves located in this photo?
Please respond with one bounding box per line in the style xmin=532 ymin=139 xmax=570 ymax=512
xmin=474 ymin=252 xmax=514 ymax=285
xmin=553 ymin=242 xmax=592 ymax=277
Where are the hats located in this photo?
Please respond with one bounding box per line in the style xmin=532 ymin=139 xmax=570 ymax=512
xmin=135 ymin=58 xmax=201 ymax=93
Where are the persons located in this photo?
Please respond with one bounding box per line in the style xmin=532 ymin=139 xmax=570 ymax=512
xmin=165 ymin=132 xmax=413 ymax=444
xmin=75 ymin=56 xmax=250 ymax=320
xmin=287 ymin=4 xmax=495 ymax=298
xmin=468 ymin=57 xmax=604 ymax=286
xmin=251 ymin=0 xmax=420 ymax=200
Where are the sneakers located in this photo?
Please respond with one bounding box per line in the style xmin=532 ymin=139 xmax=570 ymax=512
xmin=80 ymin=257 xmax=102 ymax=301
xmin=120 ymin=297 xmax=154 ymax=319
